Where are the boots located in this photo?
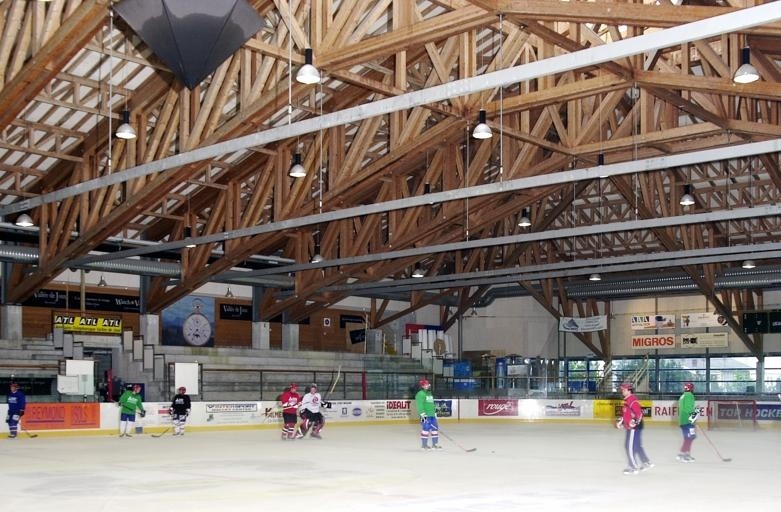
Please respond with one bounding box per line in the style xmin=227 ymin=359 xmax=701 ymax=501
xmin=282 ymin=433 xmax=323 ymax=441
xmin=420 ymin=443 xmax=443 ymax=451
xmin=622 ymin=451 xmax=697 ymax=475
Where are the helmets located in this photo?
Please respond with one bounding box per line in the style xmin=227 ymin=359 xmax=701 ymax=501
xmin=289 ymin=382 xmax=318 ymax=389
xmin=684 ymin=383 xmax=695 ymax=392
xmin=132 ymin=383 xmax=186 ymax=395
xmin=620 ymin=382 xmax=634 ymax=390
xmin=419 ymin=379 xmax=430 ymax=386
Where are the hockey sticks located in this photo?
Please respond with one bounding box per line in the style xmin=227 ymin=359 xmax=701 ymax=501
xmin=326 ymin=364 xmax=341 ymax=399
xmin=696 ymin=421 xmax=733 ymax=462
xmin=261 ymin=404 xmax=293 ymax=416
xmin=19 ymin=420 xmax=38 ymax=438
xmin=106 ymin=389 xmax=141 ymax=416
xmin=426 ymin=421 xmax=477 ymax=452
xmin=151 ymin=415 xmax=186 ymax=437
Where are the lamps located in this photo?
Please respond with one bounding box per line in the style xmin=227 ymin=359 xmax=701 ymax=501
xmin=742 ymin=261 xmax=756 ymax=269
xmin=412 ymin=261 xmax=423 ymax=279
xmin=310 ymin=226 xmax=323 ymax=264
xmin=224 ymin=287 xmax=235 ymax=298
xmin=732 ymin=0 xmax=759 ymax=83
xmin=518 ymin=208 xmax=531 ymax=226
xmin=290 ymin=137 xmax=306 ymax=177
xmin=589 ymin=273 xmax=601 ymax=280
xmin=15 ymin=174 xmax=34 ymax=227
xmin=680 ymin=185 xmax=695 ymax=205
xmin=473 ymin=25 xmax=492 ymax=139
xmin=100 ymin=275 xmax=106 ymax=289
xmin=295 ymin=0 xmax=320 ymax=85
xmin=116 ymin=61 xmax=136 ymax=140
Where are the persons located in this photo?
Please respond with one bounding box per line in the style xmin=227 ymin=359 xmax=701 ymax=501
xmin=414 ymin=379 xmax=442 ymax=450
xmin=117 ymin=384 xmax=145 ymax=438
xmin=276 ymin=382 xmax=328 ymax=441
xmin=616 ymin=383 xmax=650 ymax=473
xmin=5 ymin=383 xmax=26 ymax=438
xmin=169 ymin=386 xmax=191 ymax=435
xmin=676 ymin=383 xmax=700 ymax=463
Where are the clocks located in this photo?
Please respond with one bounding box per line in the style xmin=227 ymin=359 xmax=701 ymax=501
xmin=183 ymin=312 xmax=211 ymax=346
